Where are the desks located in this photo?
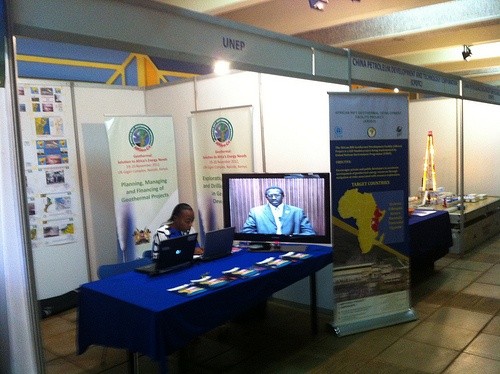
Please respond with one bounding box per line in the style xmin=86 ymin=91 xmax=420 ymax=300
xmin=408 ymin=196 xmax=498 ymax=288
xmin=76 ymin=243 xmax=334 ymax=374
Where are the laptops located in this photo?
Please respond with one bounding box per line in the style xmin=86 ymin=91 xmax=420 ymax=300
xmin=136 ymin=233 xmax=198 ymax=276
xmin=194 ymin=227 xmax=235 ymax=263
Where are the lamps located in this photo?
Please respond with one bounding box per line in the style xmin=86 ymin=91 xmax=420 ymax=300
xmin=309 ymin=0 xmax=329 ymax=11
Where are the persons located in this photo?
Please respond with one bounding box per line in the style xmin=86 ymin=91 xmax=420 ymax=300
xmin=241 ymin=186 xmax=317 ymax=236
xmin=152 ymin=203 xmax=205 ymax=263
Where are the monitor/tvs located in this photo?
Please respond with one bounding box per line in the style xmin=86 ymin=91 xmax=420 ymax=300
xmin=221 ymin=172 xmax=332 ymax=252
